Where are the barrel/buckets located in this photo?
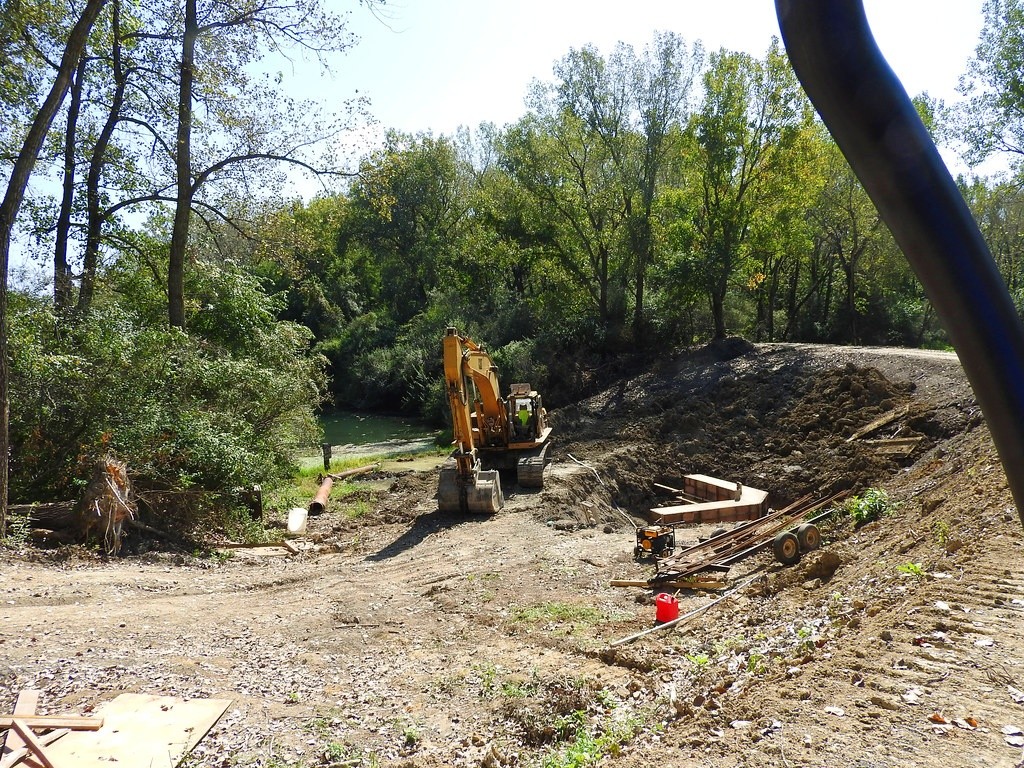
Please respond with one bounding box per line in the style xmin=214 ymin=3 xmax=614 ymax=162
xmin=287 ymin=508 xmax=308 ymax=533
xmin=655 ymin=592 xmax=679 ymax=623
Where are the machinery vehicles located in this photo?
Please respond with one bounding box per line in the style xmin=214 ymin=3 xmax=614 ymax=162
xmin=437 ymin=327 xmax=554 ymax=514
